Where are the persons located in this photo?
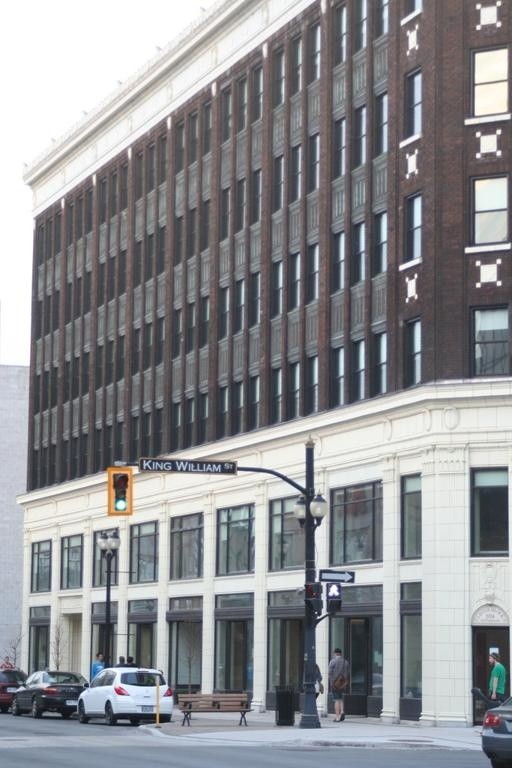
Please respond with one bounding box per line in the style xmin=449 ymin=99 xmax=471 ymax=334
xmin=328 ymin=648 xmax=350 ymax=722
xmin=0 ymin=656 xmax=14 ymax=668
xmin=315 ymin=664 xmax=322 ymax=699
xmin=487 ymin=653 xmax=506 ymax=702
xmin=92 ymin=653 xmax=105 ymax=677
xmin=127 ymin=656 xmax=137 ymax=667
xmin=115 ymin=656 xmax=127 ymax=667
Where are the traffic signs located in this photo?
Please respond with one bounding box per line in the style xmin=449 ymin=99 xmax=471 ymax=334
xmin=137 ymin=456 xmax=239 ymax=477
xmin=316 ymin=566 xmax=356 ymax=584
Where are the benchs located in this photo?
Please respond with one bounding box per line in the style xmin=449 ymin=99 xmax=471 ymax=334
xmin=178 ymin=692 xmax=252 ymax=726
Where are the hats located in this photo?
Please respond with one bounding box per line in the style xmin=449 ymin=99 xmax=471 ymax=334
xmin=491 ymin=652 xmax=500 ymax=661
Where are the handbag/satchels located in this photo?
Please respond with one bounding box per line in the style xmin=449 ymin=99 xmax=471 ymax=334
xmin=334 ymin=673 xmax=348 ymax=691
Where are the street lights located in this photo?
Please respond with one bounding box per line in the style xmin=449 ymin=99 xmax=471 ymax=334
xmin=293 ymin=436 xmax=324 ymax=731
xmin=92 ymin=524 xmax=123 ymax=673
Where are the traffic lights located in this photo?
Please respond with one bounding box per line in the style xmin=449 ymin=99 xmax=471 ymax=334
xmin=104 ymin=465 xmax=133 ymax=516
xmin=302 ymin=579 xmax=325 ymax=614
xmin=324 ymin=581 xmax=344 ymax=614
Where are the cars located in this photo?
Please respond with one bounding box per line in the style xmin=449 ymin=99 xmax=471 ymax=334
xmin=405 ymin=678 xmax=421 ymax=697
xmin=217 ymin=663 xmax=383 ymax=694
xmin=8 ymin=669 xmax=88 ymax=718
xmin=0 ymin=666 xmax=28 ymax=713
xmin=478 ymin=693 xmax=512 ymax=767
xmin=474 ymin=687 xmax=504 ymax=720
xmin=74 ymin=665 xmax=174 ymax=725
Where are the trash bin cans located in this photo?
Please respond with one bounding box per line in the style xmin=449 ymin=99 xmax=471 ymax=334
xmin=275 ymin=686 xmax=295 ymax=726
